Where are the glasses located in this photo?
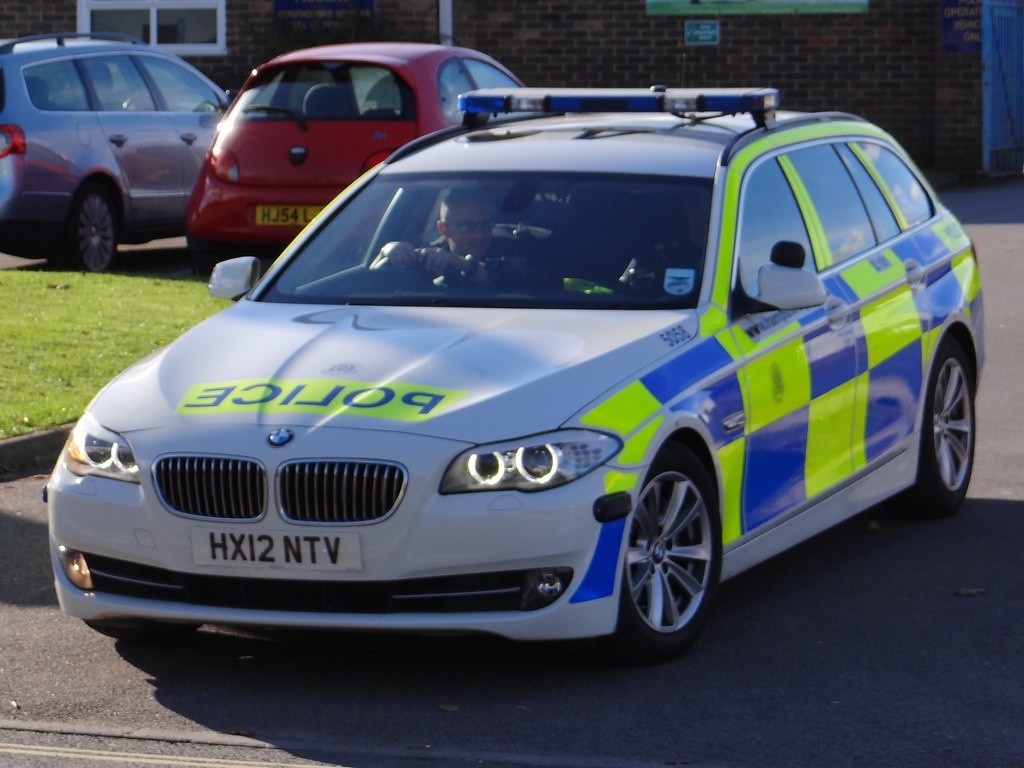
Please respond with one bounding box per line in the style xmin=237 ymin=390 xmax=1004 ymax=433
xmin=444 ymin=219 xmax=492 ymax=230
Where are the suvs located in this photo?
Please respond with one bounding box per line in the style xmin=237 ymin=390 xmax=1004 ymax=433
xmin=0 ymin=31 xmax=235 ymax=273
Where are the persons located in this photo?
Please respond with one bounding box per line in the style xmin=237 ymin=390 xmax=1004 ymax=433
xmin=367 ymin=188 xmax=522 ymax=289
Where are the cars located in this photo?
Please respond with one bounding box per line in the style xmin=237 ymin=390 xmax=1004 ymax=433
xmin=186 ymin=42 xmax=525 ymax=276
xmin=41 ymin=83 xmax=985 ymax=669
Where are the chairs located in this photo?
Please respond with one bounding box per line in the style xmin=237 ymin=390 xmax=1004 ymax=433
xmin=89 ymin=63 xmax=124 ymax=110
xmin=24 ymin=75 xmax=49 ymax=107
xmin=303 ymin=84 xmax=348 ymax=118
